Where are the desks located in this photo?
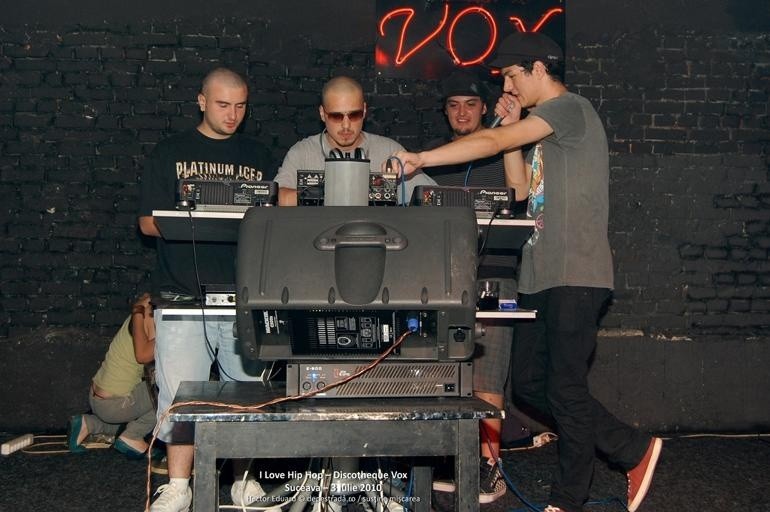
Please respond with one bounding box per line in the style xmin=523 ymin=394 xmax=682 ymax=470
xmin=169 ymin=380 xmax=501 ymax=512
xmin=148 ymin=300 xmax=536 ymax=512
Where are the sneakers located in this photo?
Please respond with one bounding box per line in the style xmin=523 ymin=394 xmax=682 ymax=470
xmin=149 ymin=483 xmax=193 ymax=512
xmin=626 ymin=437 xmax=663 ymax=512
xmin=230 ymin=476 xmax=282 ymax=512
xmin=481 ymin=457 xmax=507 ymax=503
xmin=432 ymin=478 xmax=455 ymax=495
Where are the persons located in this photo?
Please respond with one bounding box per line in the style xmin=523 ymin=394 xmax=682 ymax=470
xmin=380 ymin=41 xmax=662 ymax=511
xmin=423 ymin=70 xmax=518 ymax=504
xmin=277 ymin=75 xmax=438 ymax=207
xmin=136 ymin=66 xmax=281 ymax=512
xmin=63 ymin=295 xmax=154 ymax=464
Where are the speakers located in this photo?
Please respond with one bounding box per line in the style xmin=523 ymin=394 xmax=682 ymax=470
xmin=234 ymin=204 xmax=479 ymax=364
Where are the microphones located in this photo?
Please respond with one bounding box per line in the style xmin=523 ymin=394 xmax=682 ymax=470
xmin=488 ymin=96 xmax=515 ymax=128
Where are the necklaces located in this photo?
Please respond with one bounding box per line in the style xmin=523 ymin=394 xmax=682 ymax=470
xmin=317 ymin=125 xmax=373 ymax=161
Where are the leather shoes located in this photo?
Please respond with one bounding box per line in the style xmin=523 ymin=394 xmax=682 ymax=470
xmin=67 ymin=414 xmax=87 ymax=453
xmin=114 ymin=436 xmax=161 ymax=459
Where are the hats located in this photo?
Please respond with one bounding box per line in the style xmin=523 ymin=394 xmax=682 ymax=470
xmin=438 ymin=74 xmax=486 ymax=98
xmin=485 ymin=32 xmax=563 ymax=69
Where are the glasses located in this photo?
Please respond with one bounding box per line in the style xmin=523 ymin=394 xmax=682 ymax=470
xmin=324 ymin=108 xmax=364 ymax=123
